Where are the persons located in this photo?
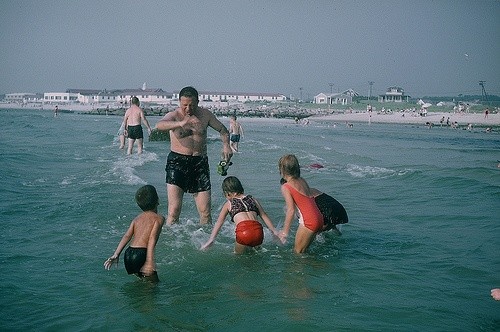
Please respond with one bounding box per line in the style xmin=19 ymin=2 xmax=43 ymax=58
xmin=347 ymin=99 xmax=500 ymax=133
xmin=199 ymin=176 xmax=287 ymax=252
xmin=278 ymin=155 xmax=324 ymax=253
xmin=118 ymin=122 xmax=128 ymax=149
xmin=103 ymin=184 xmax=166 ymax=283
xmin=122 ymin=96 xmax=152 ymax=154
xmin=280 ymin=177 xmax=349 ymax=234
xmin=105 ymin=105 xmax=110 ymax=116
xmin=227 ymin=115 xmax=244 ymax=153
xmin=54 ymin=106 xmax=58 ymax=116
xmin=155 ymin=86 xmax=233 ymax=226
xmin=295 ymin=117 xmax=311 ymax=126
